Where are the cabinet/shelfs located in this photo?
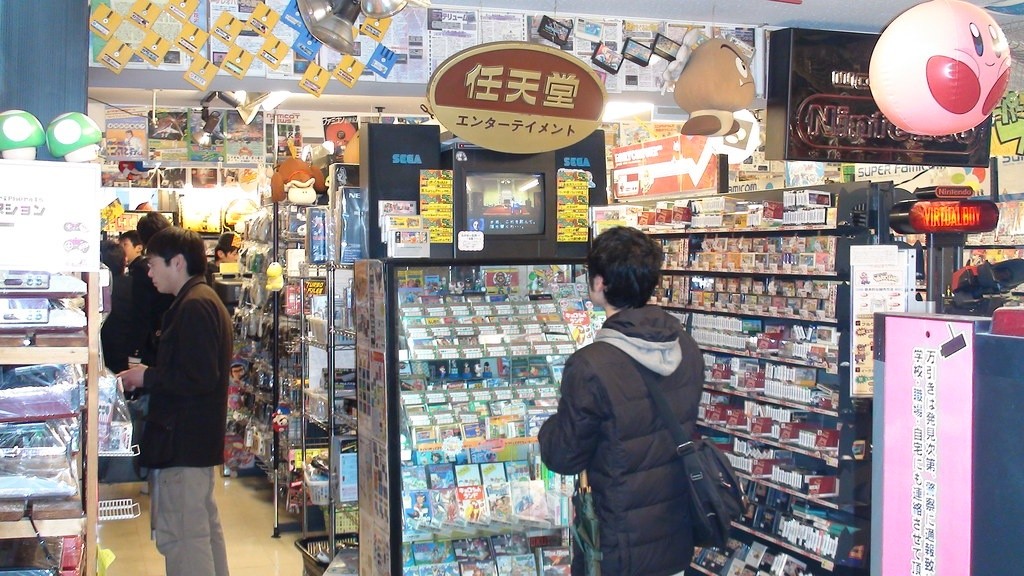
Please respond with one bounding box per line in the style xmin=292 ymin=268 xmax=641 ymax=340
xmin=0 ymin=160 xmax=1024 ymax=576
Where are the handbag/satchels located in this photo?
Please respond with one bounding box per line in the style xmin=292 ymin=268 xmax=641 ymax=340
xmin=676 ymin=437 xmax=746 ymax=542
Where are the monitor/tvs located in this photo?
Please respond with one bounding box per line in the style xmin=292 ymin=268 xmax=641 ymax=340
xmin=464 ymin=171 xmax=546 ymax=235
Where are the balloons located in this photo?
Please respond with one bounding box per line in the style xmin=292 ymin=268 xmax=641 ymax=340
xmin=867 ymin=0 xmax=1010 ymax=136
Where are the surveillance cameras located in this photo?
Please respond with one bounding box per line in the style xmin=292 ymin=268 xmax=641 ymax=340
xmin=203 ymin=116 xmax=219 ymax=133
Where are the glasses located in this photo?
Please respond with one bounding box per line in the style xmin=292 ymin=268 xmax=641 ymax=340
xmin=146 ymin=254 xmax=175 ymax=269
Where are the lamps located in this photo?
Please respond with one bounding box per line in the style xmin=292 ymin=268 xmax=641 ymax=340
xmin=218 ymin=92 xmax=261 ymax=126
xmin=297 ymin=0 xmax=432 ymax=54
xmin=191 ymin=106 xmax=221 ymax=148
xmin=242 ymin=90 xmax=272 ymax=110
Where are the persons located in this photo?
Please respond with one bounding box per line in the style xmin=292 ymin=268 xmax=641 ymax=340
xmin=100 ymin=212 xmax=177 ymax=400
xmin=205 ymin=232 xmax=238 ymax=289
xmin=125 ymin=130 xmax=143 ymax=157
xmin=116 ymin=226 xmax=234 ymax=576
xmin=537 ymin=226 xmax=705 ymax=576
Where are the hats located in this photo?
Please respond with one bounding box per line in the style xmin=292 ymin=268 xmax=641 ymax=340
xmin=219 ymin=232 xmax=241 ymax=248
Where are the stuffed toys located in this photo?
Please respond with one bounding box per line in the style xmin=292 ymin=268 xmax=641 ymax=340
xmin=271 ymin=156 xmax=325 ymax=205
xmin=265 ymin=261 xmax=284 ymax=291
xmin=674 ymin=37 xmax=756 ymax=137
xmin=273 ymin=415 xmax=289 ymax=432
